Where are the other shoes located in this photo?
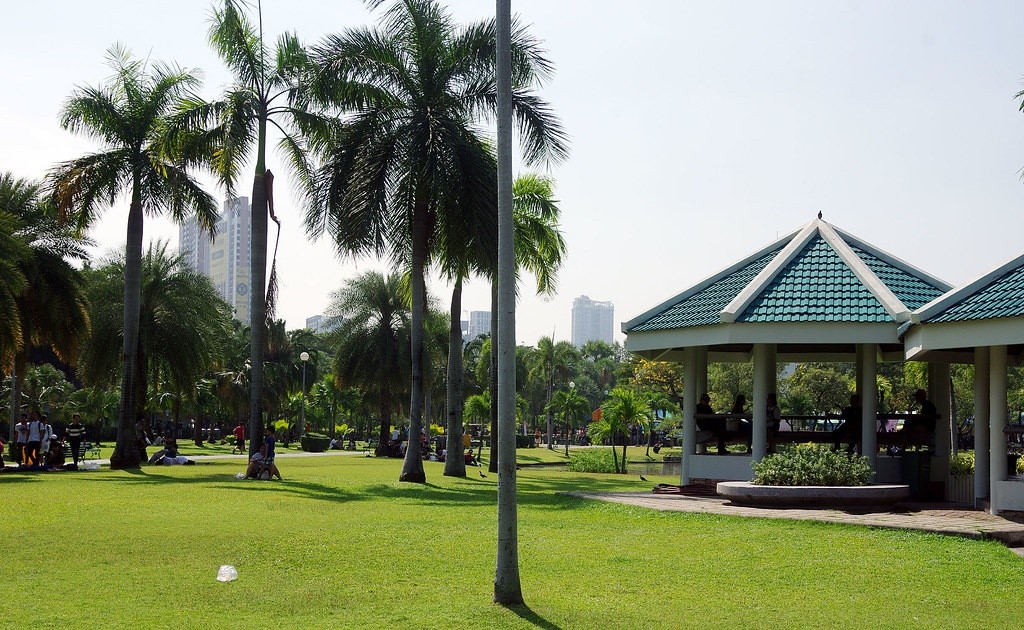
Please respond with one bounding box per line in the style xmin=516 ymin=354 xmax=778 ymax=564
xmin=267 ymin=478 xmax=272 ymax=481
xmin=718 ymin=449 xmax=730 ymax=454
xmin=474 ymin=464 xmax=479 ymax=466
xmin=256 ymin=477 xmax=260 ymax=480
xmin=278 ymin=477 xmax=282 ymax=480
xmin=241 ymin=477 xmax=248 ymax=480
xmin=233 ymin=449 xmax=235 ymax=454
xmin=240 ymin=453 xmax=243 ymax=454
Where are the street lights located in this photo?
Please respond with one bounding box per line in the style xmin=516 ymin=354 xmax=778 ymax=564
xmin=300 ymin=352 xmax=309 ymax=436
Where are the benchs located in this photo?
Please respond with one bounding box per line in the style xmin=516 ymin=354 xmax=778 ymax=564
xmin=694 ymin=413 xmax=941 ymax=456
xmin=62 ymin=446 xmax=86 ymax=465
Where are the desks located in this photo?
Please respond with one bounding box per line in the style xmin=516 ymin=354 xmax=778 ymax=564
xmin=89 ymin=449 xmax=102 ymax=459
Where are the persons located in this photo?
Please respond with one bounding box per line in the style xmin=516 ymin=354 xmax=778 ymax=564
xmin=696 ymin=393 xmax=731 ymax=456
xmin=232 ymin=422 xmax=244 ymax=454
xmin=895 ymin=389 xmax=936 ymax=456
xmin=731 ymin=394 xmax=752 ymax=454
xmin=15 ymin=411 xmax=66 ymax=472
xmin=467 ymin=425 xmax=574 ymax=445
xmin=280 ymin=421 xmax=380 ymax=451
xmin=135 ymin=418 xmax=227 ymax=465
xmin=766 ymin=393 xmax=781 ymax=454
xmin=61 ymin=413 xmax=87 ymax=470
xmin=574 ymin=424 xmax=674 ymax=447
xmin=464 ymin=450 xmax=478 ymax=465
xmin=389 ymin=425 xmax=445 ymax=462
xmin=242 ymin=425 xmax=282 ymax=481
xmin=830 ymin=393 xmax=862 ymax=454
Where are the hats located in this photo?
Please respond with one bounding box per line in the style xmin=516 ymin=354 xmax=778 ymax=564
xmin=911 ymin=390 xmax=926 ymax=397
xmin=41 ymin=415 xmax=48 ymax=420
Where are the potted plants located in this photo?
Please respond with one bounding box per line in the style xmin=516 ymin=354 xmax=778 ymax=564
xmin=947 ymin=455 xmax=974 ymax=503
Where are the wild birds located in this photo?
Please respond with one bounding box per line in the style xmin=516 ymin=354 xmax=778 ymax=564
xmin=479 ymin=471 xmax=487 ymax=479
xmin=640 ymin=475 xmax=647 ymax=481
